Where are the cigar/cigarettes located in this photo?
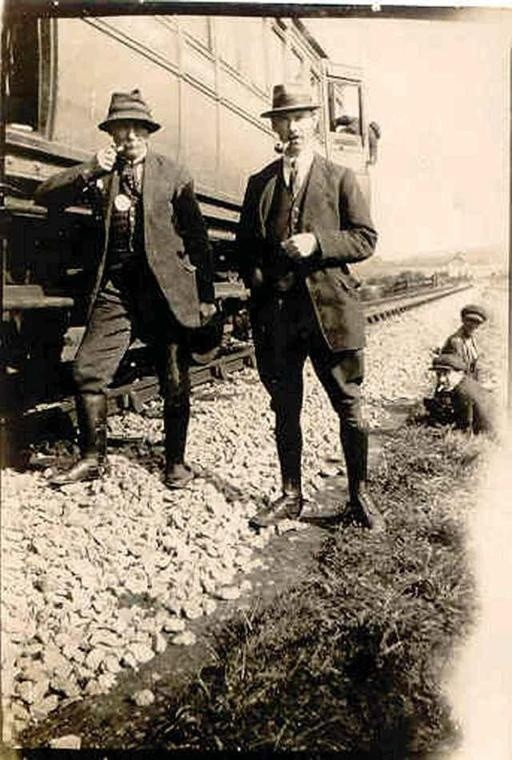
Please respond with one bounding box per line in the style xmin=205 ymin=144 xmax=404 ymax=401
xmin=115 ymin=145 xmax=125 ymax=153
xmin=437 ymin=384 xmax=444 ymax=392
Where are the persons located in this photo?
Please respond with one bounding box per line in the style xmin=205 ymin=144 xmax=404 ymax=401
xmin=234 ymin=81 xmax=391 ymax=529
xmin=425 ymin=354 xmax=502 ymax=438
xmin=437 ymin=305 xmax=488 ymax=369
xmin=29 ymin=91 xmax=218 ymax=489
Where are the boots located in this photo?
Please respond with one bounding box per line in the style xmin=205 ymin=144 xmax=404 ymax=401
xmin=162 ymin=406 xmax=192 ymax=486
xmin=51 ymin=390 xmax=108 ymax=485
xmin=341 ymin=433 xmax=383 ymax=530
xmin=249 ymin=434 xmax=303 ymax=527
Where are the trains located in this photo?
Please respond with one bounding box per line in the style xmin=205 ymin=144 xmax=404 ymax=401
xmin=0 ymin=1 xmax=388 ymax=428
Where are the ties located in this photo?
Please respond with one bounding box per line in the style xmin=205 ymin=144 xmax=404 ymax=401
xmin=289 ymin=161 xmax=298 ymax=195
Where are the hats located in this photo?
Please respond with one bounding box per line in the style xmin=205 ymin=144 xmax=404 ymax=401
xmin=98 ymin=89 xmax=162 ymax=134
xmin=260 ymin=83 xmax=321 ymax=117
xmin=461 ymin=304 xmax=488 ymax=322
xmin=428 ymin=354 xmax=468 ymax=370
